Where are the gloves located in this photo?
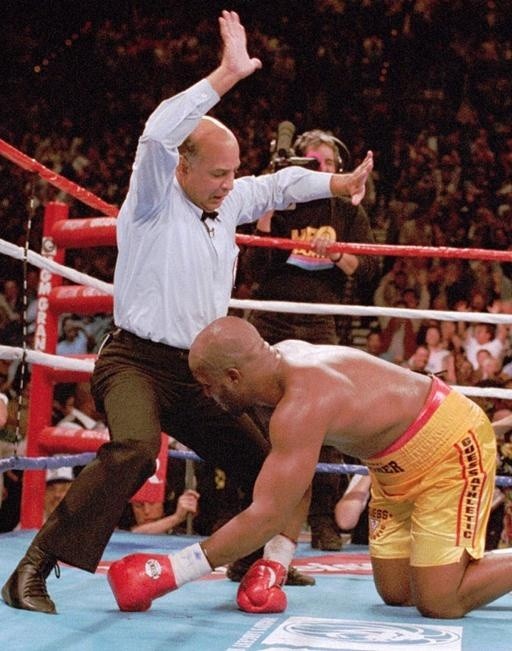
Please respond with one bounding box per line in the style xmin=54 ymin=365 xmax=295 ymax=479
xmin=106 ymin=542 xmax=215 ymax=612
xmin=237 ymin=534 xmax=297 ymax=613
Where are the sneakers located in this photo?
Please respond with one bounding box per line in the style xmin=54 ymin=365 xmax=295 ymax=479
xmin=309 ymin=516 xmax=342 ymax=550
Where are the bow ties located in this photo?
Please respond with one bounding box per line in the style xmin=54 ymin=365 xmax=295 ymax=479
xmin=201 ymin=210 xmax=218 ymax=222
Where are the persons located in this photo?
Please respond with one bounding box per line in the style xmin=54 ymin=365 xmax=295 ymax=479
xmin=108 ymin=317 xmax=511 ymax=619
xmin=1 ymin=1 xmax=512 ymax=538
xmin=1 ymin=6 xmax=379 ymax=616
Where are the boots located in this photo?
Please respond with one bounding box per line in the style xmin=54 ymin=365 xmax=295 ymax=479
xmin=0 ymin=545 xmax=60 ymax=614
xmin=227 ymin=548 xmax=313 ymax=587
